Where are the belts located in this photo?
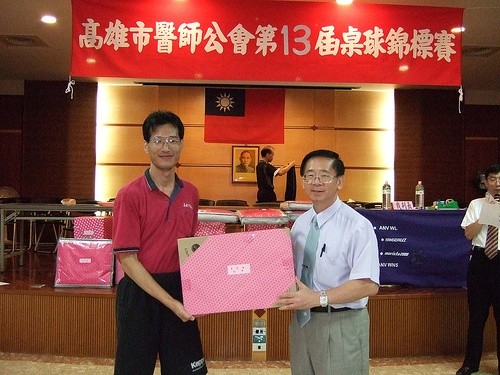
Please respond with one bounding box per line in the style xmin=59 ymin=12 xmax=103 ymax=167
xmin=474 ymin=247 xmax=500 ymax=254
xmin=309 ymin=305 xmax=352 ymax=312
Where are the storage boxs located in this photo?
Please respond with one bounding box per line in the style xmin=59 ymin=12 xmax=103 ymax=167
xmin=72 ymin=215 xmax=112 ymax=239
xmin=177 ymin=227 xmax=297 ymax=317
xmin=55 ymin=237 xmax=114 ymax=288
xmin=242 ymin=221 xmax=290 ymax=231
xmin=195 ymin=221 xmax=226 ymax=238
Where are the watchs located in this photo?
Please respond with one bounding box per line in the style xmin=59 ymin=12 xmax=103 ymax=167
xmin=319 ymin=290 xmax=328 ymax=308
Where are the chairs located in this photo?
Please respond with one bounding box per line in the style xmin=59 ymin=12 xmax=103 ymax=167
xmin=0 ymin=196 xmax=117 ymax=254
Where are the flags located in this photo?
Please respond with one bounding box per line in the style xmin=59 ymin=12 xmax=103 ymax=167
xmin=204 ymin=87 xmax=285 ymax=145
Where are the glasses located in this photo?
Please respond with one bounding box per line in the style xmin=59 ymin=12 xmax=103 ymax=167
xmin=301 ymin=174 xmax=339 ymax=183
xmin=149 ymin=135 xmax=181 ymax=146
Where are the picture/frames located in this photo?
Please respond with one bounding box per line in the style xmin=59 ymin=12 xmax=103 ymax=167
xmin=231 ymin=145 xmax=260 ymax=183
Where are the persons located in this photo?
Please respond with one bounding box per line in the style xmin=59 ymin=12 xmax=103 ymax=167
xmin=236 ymin=150 xmax=255 ymax=173
xmin=256 ymin=145 xmax=296 ymax=202
xmin=111 ymin=110 xmax=208 ymax=375
xmin=455 ymin=164 xmax=500 ymax=375
xmin=276 ymin=150 xmax=380 ymax=374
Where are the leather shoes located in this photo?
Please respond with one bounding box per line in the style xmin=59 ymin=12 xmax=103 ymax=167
xmin=456 ymin=365 xmax=479 ymax=375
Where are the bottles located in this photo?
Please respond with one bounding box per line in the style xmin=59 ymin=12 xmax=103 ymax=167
xmin=382 ymin=181 xmax=392 ymax=210
xmin=415 ymin=181 xmax=425 ymax=210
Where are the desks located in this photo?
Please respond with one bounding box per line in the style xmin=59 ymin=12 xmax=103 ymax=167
xmin=0 ymin=203 xmax=114 ymax=272
xmin=356 ymin=208 xmax=468 ymax=286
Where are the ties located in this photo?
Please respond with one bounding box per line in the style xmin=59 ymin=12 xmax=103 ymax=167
xmin=485 ymin=225 xmax=498 ymax=259
xmin=297 ymin=216 xmax=320 ymax=328
